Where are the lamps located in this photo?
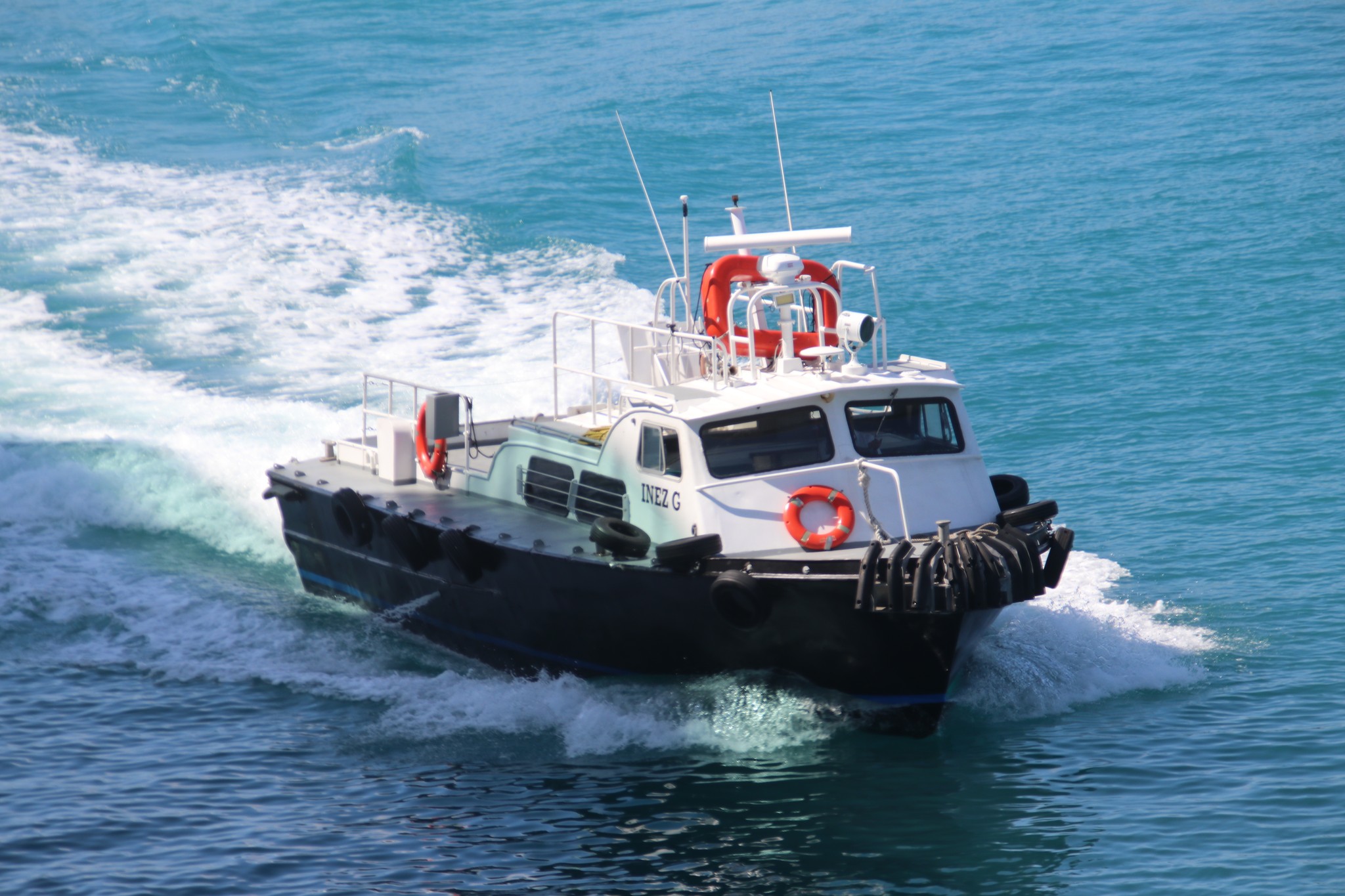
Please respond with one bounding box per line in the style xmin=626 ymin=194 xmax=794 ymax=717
xmin=837 ymin=305 xmax=875 ymax=375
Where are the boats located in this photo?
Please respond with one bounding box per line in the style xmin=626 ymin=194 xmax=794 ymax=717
xmin=260 ymin=88 xmax=1076 ymax=744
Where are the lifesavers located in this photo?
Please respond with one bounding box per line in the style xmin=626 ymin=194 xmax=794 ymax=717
xmin=784 ymin=485 xmax=855 ymax=551
xmin=989 ymin=474 xmax=1030 ymax=511
xmin=995 ymin=499 xmax=1059 ymax=529
xmin=329 ymin=487 xmax=375 ymax=548
xmin=655 ymin=533 xmax=723 ymax=566
xmin=439 ymin=528 xmax=483 ymax=583
xmin=709 ymin=570 xmax=763 ymax=630
xmin=416 ymin=400 xmax=448 ymax=481
xmin=382 ymin=515 xmax=434 ymax=569
xmin=590 ymin=517 xmax=650 ymax=557
xmin=1043 ymin=526 xmax=1075 ymax=589
